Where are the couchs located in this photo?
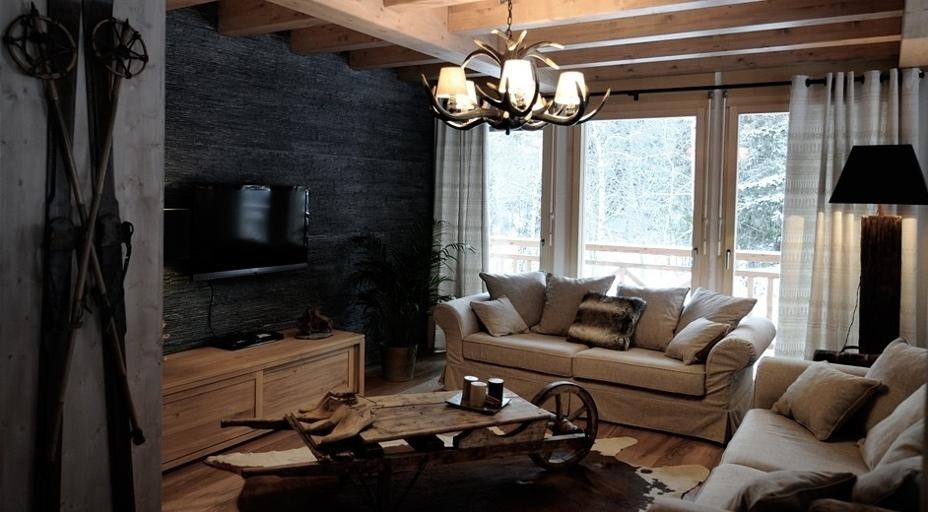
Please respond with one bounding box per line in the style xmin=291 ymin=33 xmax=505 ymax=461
xmin=432 ymin=293 xmax=776 ymax=449
xmin=646 ymin=358 xmax=928 ymax=512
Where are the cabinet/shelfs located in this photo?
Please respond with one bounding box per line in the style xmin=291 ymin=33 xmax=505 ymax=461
xmin=158 ymin=327 xmax=366 ymax=473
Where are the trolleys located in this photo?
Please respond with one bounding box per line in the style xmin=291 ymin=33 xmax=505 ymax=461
xmin=201 ymin=380 xmax=600 ymax=489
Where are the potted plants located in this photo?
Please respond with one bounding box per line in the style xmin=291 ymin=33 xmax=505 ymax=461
xmin=342 ymin=212 xmax=476 ymax=383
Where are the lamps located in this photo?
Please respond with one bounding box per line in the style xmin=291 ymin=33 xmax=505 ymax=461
xmin=828 ymin=144 xmax=928 ymax=356
xmin=421 ymin=0 xmax=611 ymax=136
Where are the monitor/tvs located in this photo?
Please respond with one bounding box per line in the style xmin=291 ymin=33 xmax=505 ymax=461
xmin=188 ymin=177 xmax=311 ymax=283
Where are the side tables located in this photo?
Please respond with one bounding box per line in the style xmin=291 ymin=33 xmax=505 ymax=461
xmin=813 ymin=350 xmax=880 ymax=367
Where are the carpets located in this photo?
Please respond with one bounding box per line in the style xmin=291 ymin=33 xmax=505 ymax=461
xmin=203 ymin=423 xmax=710 ymax=512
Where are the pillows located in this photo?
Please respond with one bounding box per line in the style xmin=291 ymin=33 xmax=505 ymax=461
xmin=849 ymin=336 xmax=928 ymax=512
xmin=530 ymin=272 xmax=614 ymax=336
xmin=478 ymin=268 xmax=547 ymax=327
xmin=771 ymin=359 xmax=882 ymax=440
xmin=618 ymin=286 xmax=689 ymax=351
xmin=720 ymin=470 xmax=857 ymax=512
xmin=470 ymin=294 xmax=530 ymax=338
xmin=567 ymin=289 xmax=647 ymax=349
xmin=673 ymin=284 xmax=758 ymax=344
xmin=665 ymin=316 xmax=730 ymax=363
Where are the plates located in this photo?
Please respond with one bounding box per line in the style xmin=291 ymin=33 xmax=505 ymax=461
xmin=443 ymin=391 xmax=511 ymax=416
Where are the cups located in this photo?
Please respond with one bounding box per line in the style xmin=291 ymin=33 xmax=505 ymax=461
xmin=461 ymin=375 xmax=505 ymax=408
